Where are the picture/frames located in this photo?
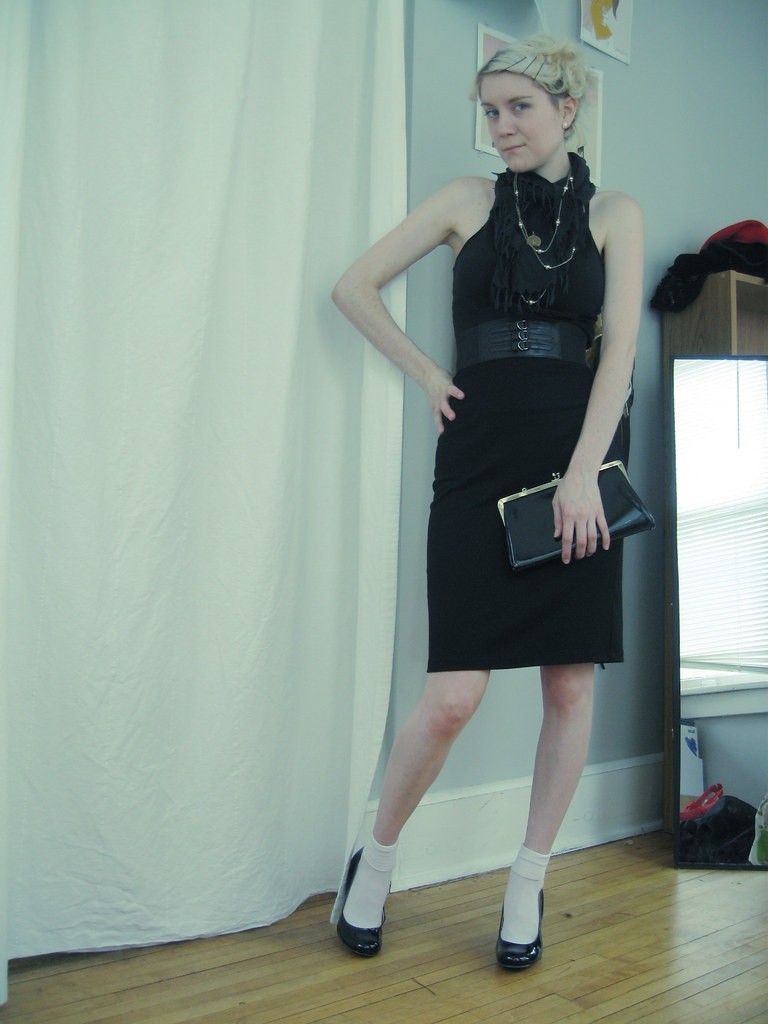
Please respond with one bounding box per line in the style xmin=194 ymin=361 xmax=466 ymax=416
xmin=579 ymin=0 xmax=632 ymax=65
xmin=474 ymin=23 xmax=603 ymax=185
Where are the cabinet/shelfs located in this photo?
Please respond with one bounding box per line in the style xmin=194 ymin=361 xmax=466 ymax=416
xmin=657 ymin=269 xmax=768 ymax=835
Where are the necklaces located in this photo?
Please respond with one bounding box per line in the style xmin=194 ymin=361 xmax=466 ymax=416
xmin=513 ymin=172 xmax=579 ymax=305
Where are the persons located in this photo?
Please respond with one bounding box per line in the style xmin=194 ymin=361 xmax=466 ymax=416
xmin=331 ymin=37 xmax=643 ymax=970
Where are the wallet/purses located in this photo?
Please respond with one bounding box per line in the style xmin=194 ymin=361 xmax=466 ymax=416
xmin=495 ymin=459 xmax=657 ymax=572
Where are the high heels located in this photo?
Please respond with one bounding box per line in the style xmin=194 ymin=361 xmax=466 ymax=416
xmin=496 ymin=888 xmax=544 ymax=970
xmin=337 ymin=847 xmax=392 ymax=956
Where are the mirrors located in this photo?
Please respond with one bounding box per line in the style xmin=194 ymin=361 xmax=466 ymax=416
xmin=666 ymin=353 xmax=768 ymax=876
